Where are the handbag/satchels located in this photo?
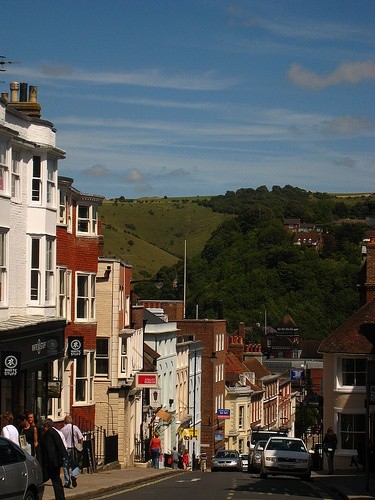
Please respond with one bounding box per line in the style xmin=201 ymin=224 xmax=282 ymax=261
xmin=73 ymin=440 xmax=83 ymax=451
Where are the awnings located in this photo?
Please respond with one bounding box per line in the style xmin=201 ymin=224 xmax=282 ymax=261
xmin=155 ymin=408 xmax=172 ymax=422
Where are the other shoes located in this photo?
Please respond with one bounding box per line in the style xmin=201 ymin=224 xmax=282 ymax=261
xmin=71 ymin=475 xmax=78 ymax=488
xmin=64 ymin=484 xmax=70 ymax=488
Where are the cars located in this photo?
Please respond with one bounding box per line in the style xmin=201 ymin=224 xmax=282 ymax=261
xmin=249 ymin=440 xmax=286 ymax=474
xmin=0 ymin=435 xmax=44 ymax=500
xmin=239 ymin=454 xmax=249 ymax=472
xmin=210 ymin=450 xmax=242 ymax=472
xmin=257 ymin=436 xmax=316 ymax=482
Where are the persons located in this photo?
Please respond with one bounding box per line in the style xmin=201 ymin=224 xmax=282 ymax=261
xmin=200 ymin=454 xmax=207 ymax=472
xmin=0 ymin=410 xmax=38 ymax=456
xmin=171 ymin=447 xmax=189 ymax=471
xmin=60 ymin=415 xmax=83 ymax=488
xmin=322 ymin=427 xmax=338 ymax=474
xmin=38 ymin=418 xmax=69 ymax=500
xmin=150 ymin=431 xmax=162 ymax=469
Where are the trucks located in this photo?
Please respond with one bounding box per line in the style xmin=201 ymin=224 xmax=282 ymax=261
xmin=247 ymin=429 xmax=290 ymax=473
